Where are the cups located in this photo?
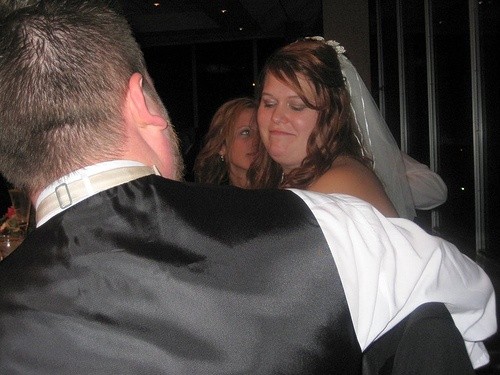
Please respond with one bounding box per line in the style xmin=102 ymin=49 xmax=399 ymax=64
xmin=9 ymin=189 xmax=31 ymax=236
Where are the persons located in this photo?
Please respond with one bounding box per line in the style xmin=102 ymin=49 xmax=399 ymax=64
xmin=0 ymin=0 xmax=497 ymax=375
xmin=246 ymin=35 xmax=417 ymax=221
xmin=193 ymin=98 xmax=259 ymax=190
